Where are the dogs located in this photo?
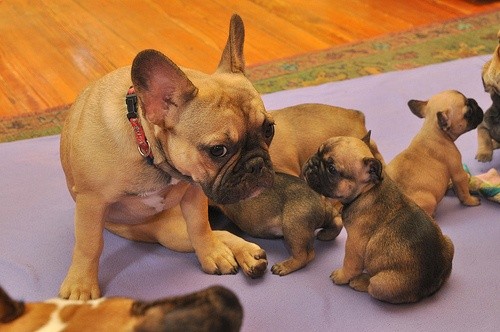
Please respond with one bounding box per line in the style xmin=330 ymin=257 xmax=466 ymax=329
xmin=0 ymin=283 xmax=245 ymax=332
xmin=475 ymin=29 xmax=500 ymax=161
xmin=303 ymin=130 xmax=454 ymax=305
xmin=56 ymin=13 xmax=385 ymax=301
xmin=386 ymin=90 xmax=484 ymax=221
